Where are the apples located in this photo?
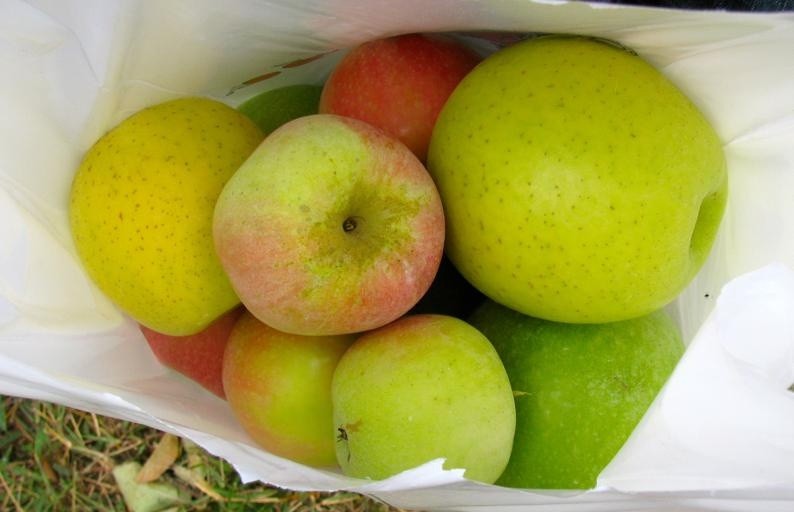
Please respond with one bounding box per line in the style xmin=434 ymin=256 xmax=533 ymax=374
xmin=211 ymin=112 xmax=446 ymax=336
xmin=467 ymin=299 xmax=687 ymax=490
xmin=329 ymin=314 xmax=517 ymax=486
xmin=236 ymin=84 xmax=324 ymax=138
xmin=319 ymin=34 xmax=481 ymax=164
xmin=220 ymin=310 xmax=358 ymax=471
xmin=138 ymin=303 xmax=246 ymax=402
xmin=67 ymin=96 xmax=266 ymax=337
xmin=427 ymin=36 xmax=729 ymax=324
